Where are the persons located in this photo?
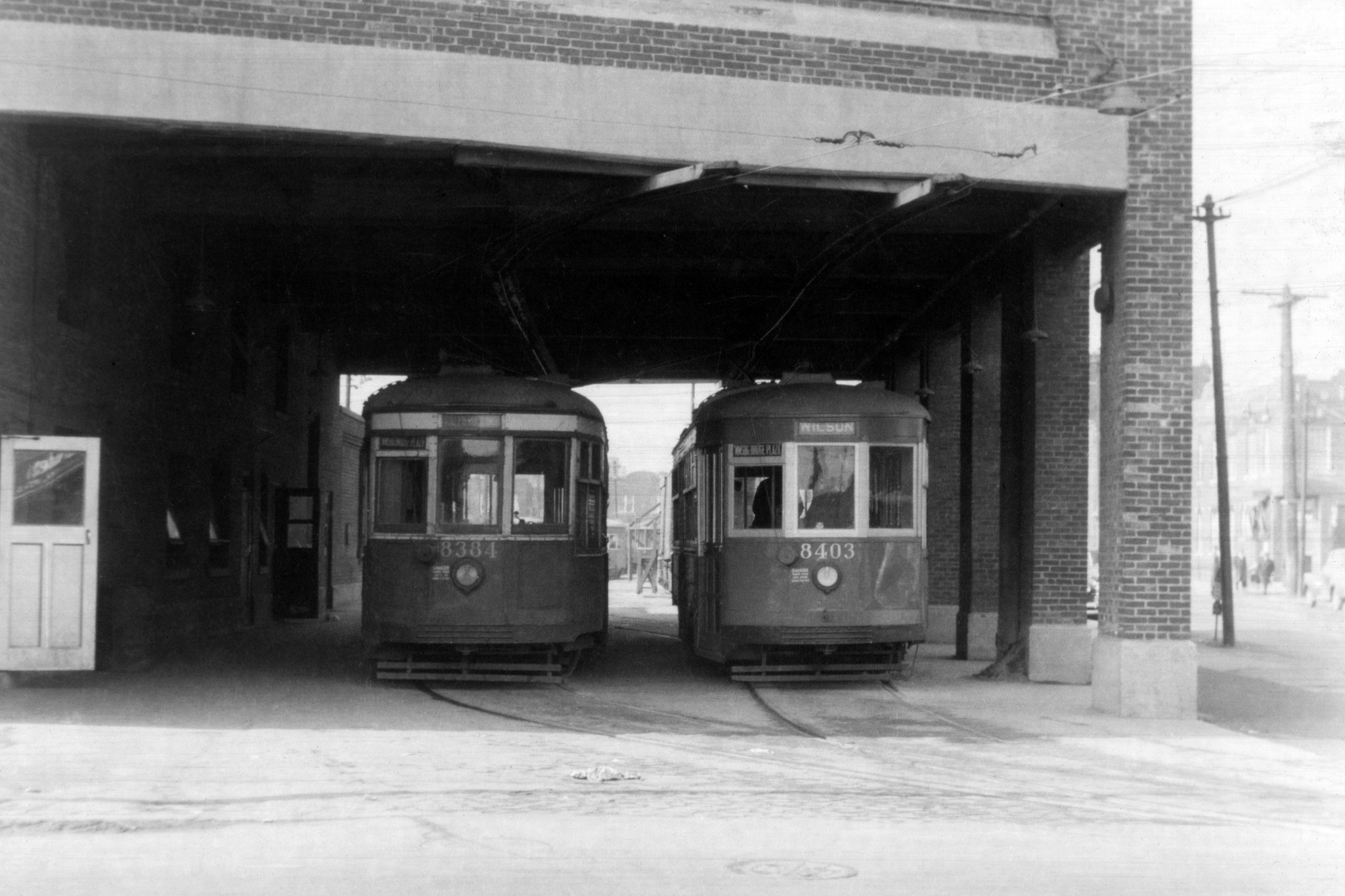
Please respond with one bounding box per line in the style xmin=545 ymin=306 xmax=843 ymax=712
xmin=1214 ymin=554 xmax=1274 ymax=590
xmin=750 ymin=467 xmax=804 ymax=529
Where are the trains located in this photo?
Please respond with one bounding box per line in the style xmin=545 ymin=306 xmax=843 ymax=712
xmin=355 ymin=356 xmax=611 ymax=687
xmin=669 ymin=369 xmax=931 ymax=680
xmin=606 ymin=518 xmax=630 ymax=581
